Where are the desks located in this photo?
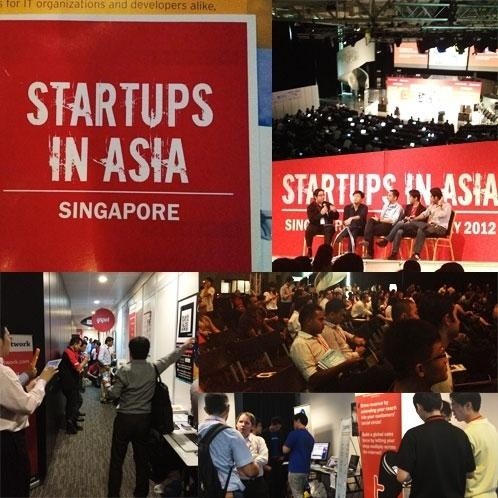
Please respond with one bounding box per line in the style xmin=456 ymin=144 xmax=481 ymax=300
xmin=307 ymin=464 xmax=362 ymax=498
xmin=163 ymin=410 xmax=198 ymax=498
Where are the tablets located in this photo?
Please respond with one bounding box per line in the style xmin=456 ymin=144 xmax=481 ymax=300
xmin=45 ymin=359 xmax=62 ymax=367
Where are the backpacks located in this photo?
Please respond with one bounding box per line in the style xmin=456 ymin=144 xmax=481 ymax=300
xmin=198 ymin=423 xmax=234 ymax=498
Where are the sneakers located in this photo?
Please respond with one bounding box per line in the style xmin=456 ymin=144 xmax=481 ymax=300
xmin=359 ymin=236 xmax=421 ymax=261
xmin=66 ymin=412 xmax=85 ymax=435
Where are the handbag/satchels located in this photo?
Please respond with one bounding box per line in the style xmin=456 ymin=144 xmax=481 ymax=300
xmin=152 ymin=381 xmax=175 ymax=436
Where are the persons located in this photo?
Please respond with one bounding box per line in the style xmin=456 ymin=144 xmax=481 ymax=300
xmin=0 ymin=105 xmax=498 ymax=498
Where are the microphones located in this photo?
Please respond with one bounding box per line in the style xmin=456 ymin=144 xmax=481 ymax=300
xmin=322 ymin=203 xmax=329 ymax=215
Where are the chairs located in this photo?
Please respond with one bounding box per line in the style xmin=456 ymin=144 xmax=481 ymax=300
xmin=198 ymin=318 xmax=303 ymax=393
xmin=303 ymin=209 xmax=456 ymax=261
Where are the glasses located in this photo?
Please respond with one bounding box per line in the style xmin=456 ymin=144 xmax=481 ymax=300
xmin=419 ymin=344 xmax=446 ymax=369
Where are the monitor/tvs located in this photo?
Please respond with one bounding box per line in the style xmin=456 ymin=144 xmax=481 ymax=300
xmin=349 ymin=455 xmax=359 ymax=471
xmin=310 ymin=442 xmax=330 ymax=462
